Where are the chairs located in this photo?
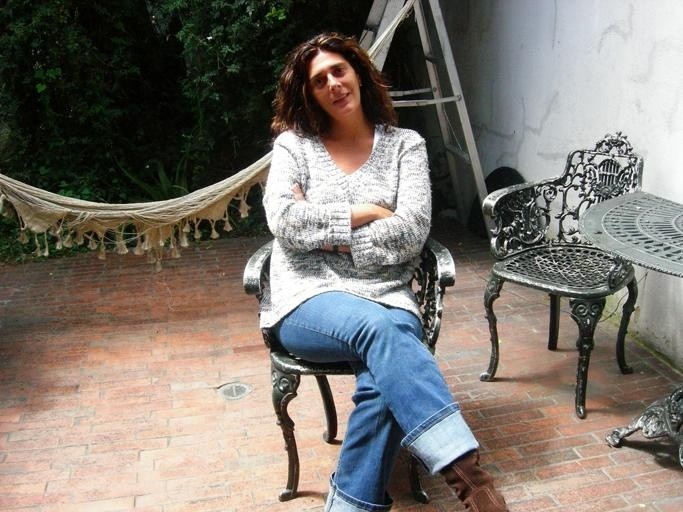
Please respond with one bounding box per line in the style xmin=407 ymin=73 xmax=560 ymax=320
xmin=242 ymin=233 xmax=457 ymax=504
xmin=479 ymin=131 xmax=644 ymax=420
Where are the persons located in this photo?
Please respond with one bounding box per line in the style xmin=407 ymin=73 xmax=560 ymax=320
xmin=258 ymin=30 xmax=508 ymax=512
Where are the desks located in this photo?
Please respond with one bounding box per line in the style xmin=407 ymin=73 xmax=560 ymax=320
xmin=578 ymin=190 xmax=683 ymax=471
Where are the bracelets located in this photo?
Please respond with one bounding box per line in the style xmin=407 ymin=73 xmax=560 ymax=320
xmin=334 ymin=245 xmax=339 ymax=255
